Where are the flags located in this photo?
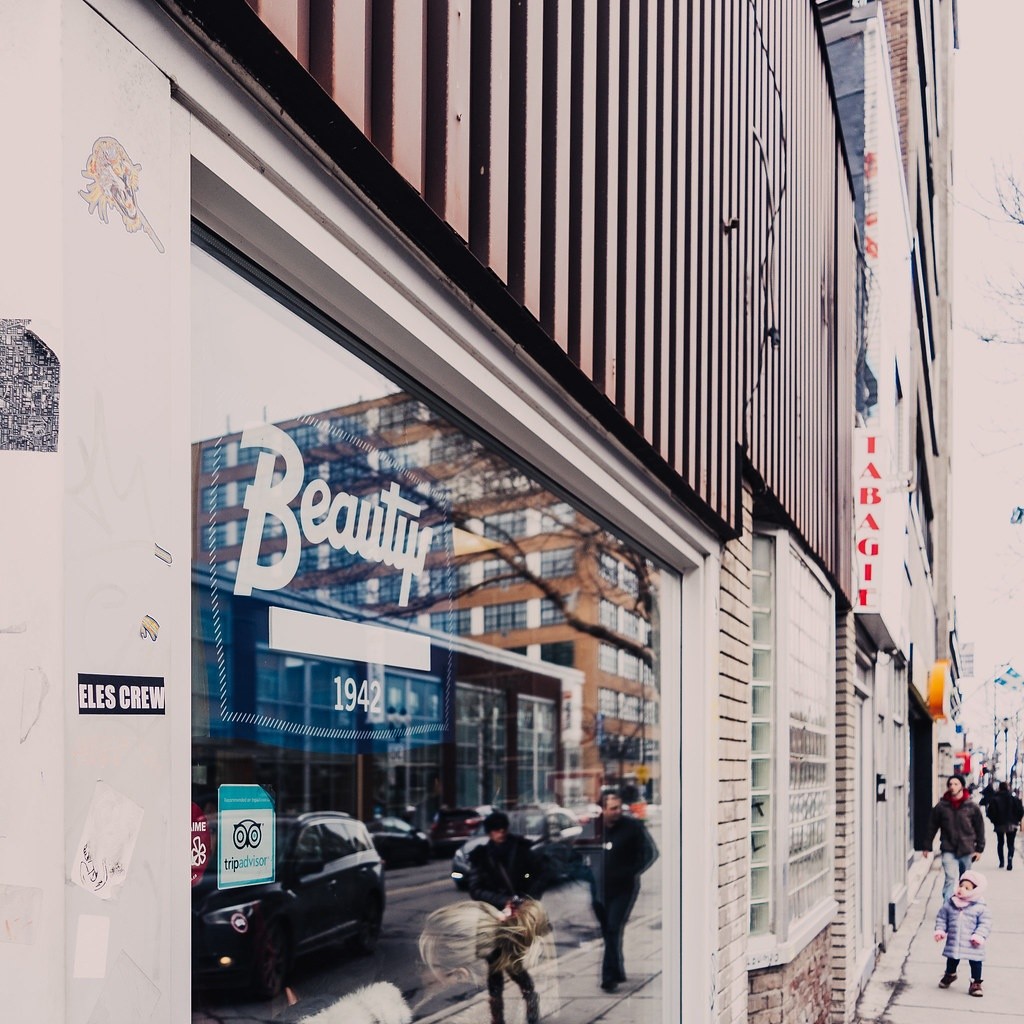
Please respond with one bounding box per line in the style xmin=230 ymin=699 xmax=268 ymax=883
xmin=993 ymin=668 xmax=1024 ymax=691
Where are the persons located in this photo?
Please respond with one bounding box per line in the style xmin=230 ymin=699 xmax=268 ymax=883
xmin=923 ymin=775 xmax=985 ymax=905
xmin=267 ymin=899 xmax=561 ymax=1024
xmin=934 ymin=869 xmax=993 ymax=997
xmin=574 ymin=790 xmax=661 ymax=992
xmin=986 ymin=782 xmax=1024 ymax=870
xmin=967 ymin=783 xmax=996 ymax=816
xmin=468 ymin=813 xmax=546 ymax=1024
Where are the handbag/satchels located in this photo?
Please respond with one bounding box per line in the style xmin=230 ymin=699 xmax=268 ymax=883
xmin=1009 ymin=823 xmax=1022 ymax=833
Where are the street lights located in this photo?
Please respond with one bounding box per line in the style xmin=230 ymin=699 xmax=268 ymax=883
xmin=1003 ymin=717 xmax=1010 ymax=781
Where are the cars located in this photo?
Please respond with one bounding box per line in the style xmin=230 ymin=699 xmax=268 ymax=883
xmin=191 ymin=808 xmax=385 ymax=1002
xmin=426 ymin=806 xmax=494 ymax=858
xmin=451 ymin=807 xmax=582 ymax=897
xmin=361 ymin=817 xmax=431 ymax=867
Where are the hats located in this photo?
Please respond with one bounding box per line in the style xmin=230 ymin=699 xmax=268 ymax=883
xmin=483 ymin=812 xmax=509 ymax=830
xmin=960 ymin=869 xmax=983 ymax=887
xmin=947 ymin=775 xmax=966 ymax=787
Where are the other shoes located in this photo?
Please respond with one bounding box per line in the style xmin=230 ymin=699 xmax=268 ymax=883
xmin=999 ymin=859 xmax=1005 ymax=867
xmin=1007 ymin=858 xmax=1012 ymax=871
xmin=968 ymin=981 xmax=983 ymax=997
xmin=938 ymin=972 xmax=958 ymax=988
xmin=490 ymin=1019 xmax=505 ymax=1024
xmin=523 ymin=993 xmax=540 ymax=1024
